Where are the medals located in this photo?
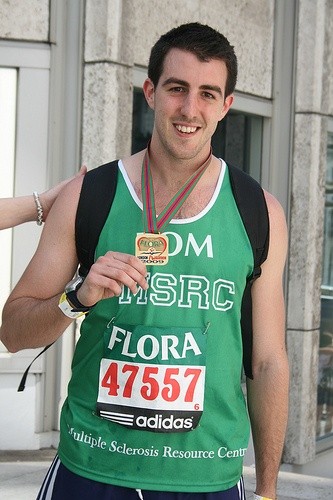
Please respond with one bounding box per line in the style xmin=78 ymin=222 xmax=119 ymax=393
xmin=135 ymin=232 xmax=169 ymax=266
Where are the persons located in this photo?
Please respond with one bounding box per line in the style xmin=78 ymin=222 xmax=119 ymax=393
xmin=0 ymin=165 xmax=88 ymax=230
xmin=0 ymin=21 xmax=291 ymax=500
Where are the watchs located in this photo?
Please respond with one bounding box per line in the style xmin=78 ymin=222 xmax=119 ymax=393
xmin=64 ymin=277 xmax=99 ymax=312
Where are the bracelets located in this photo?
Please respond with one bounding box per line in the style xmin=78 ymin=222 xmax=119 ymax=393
xmin=58 ymin=292 xmax=89 ymax=319
xmin=253 ymin=492 xmax=273 ymax=500
xmin=34 ymin=192 xmax=43 ymax=227
